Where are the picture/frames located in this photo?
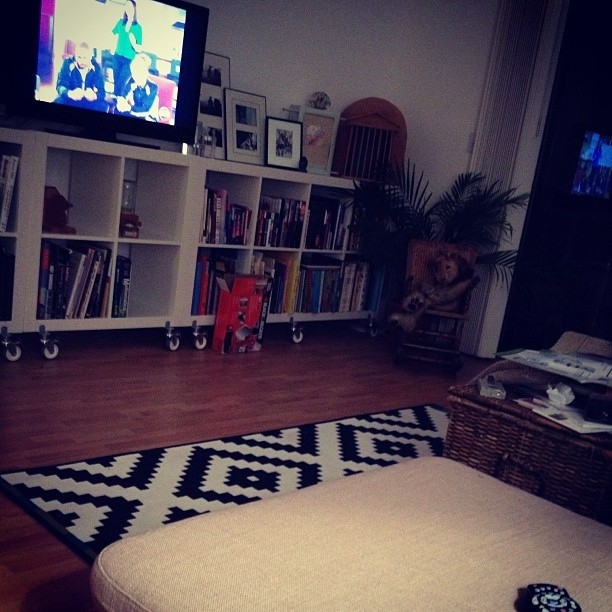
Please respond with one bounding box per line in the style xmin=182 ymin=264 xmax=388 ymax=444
xmin=224 ymin=86 xmax=266 ymax=163
xmin=197 ymin=52 xmax=230 ymax=160
xmin=264 ymin=117 xmax=304 ymax=171
xmin=297 ymin=105 xmax=340 ymax=176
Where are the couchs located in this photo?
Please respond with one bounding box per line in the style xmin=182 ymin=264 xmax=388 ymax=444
xmin=90 ymin=454 xmax=612 ymax=612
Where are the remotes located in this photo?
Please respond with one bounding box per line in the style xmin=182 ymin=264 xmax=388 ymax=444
xmin=527 ymin=584 xmax=581 ymax=612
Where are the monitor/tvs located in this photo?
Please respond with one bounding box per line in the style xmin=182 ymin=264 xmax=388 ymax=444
xmin=567 ymin=122 xmax=612 ymax=205
xmin=0 ymin=0 xmax=211 ymax=150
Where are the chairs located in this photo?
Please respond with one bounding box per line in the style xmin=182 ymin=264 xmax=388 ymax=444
xmin=394 ymin=237 xmax=476 ymax=370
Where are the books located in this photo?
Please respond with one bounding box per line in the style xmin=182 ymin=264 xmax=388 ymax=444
xmin=0 ymin=155 xmax=18 ymax=232
xmin=35 ymin=240 xmax=131 ymax=318
xmin=0 ymin=245 xmax=15 ymax=321
xmin=191 ymin=186 xmax=384 ymax=316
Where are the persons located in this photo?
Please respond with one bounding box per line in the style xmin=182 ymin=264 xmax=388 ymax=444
xmin=54 ymin=41 xmax=108 ymax=112
xmin=112 ymin=1 xmax=143 ymax=61
xmin=114 ymin=53 xmax=160 ymax=122
xmin=386 ymin=249 xmax=481 ymax=334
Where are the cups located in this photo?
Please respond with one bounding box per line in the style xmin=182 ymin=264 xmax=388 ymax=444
xmin=203 ymin=145 xmax=211 ymax=157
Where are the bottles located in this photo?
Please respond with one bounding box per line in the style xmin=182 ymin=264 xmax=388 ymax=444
xmin=212 ymin=130 xmax=217 ymax=158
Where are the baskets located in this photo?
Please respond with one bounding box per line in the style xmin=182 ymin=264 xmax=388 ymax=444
xmin=439 ymin=382 xmax=612 ymax=525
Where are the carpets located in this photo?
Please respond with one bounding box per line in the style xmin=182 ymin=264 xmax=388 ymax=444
xmin=0 ymin=402 xmax=449 ymax=566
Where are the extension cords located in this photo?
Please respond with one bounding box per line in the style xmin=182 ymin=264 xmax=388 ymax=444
xmin=478 ymin=378 xmax=506 ymax=399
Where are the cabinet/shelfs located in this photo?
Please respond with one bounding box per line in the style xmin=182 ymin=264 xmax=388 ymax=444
xmin=2 ymin=128 xmax=36 ymax=360
xmin=186 ymin=155 xmax=379 ymax=352
xmin=26 ymin=133 xmax=198 ymax=360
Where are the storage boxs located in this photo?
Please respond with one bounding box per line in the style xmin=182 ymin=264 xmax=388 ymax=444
xmin=215 ymin=270 xmax=273 ymax=353
